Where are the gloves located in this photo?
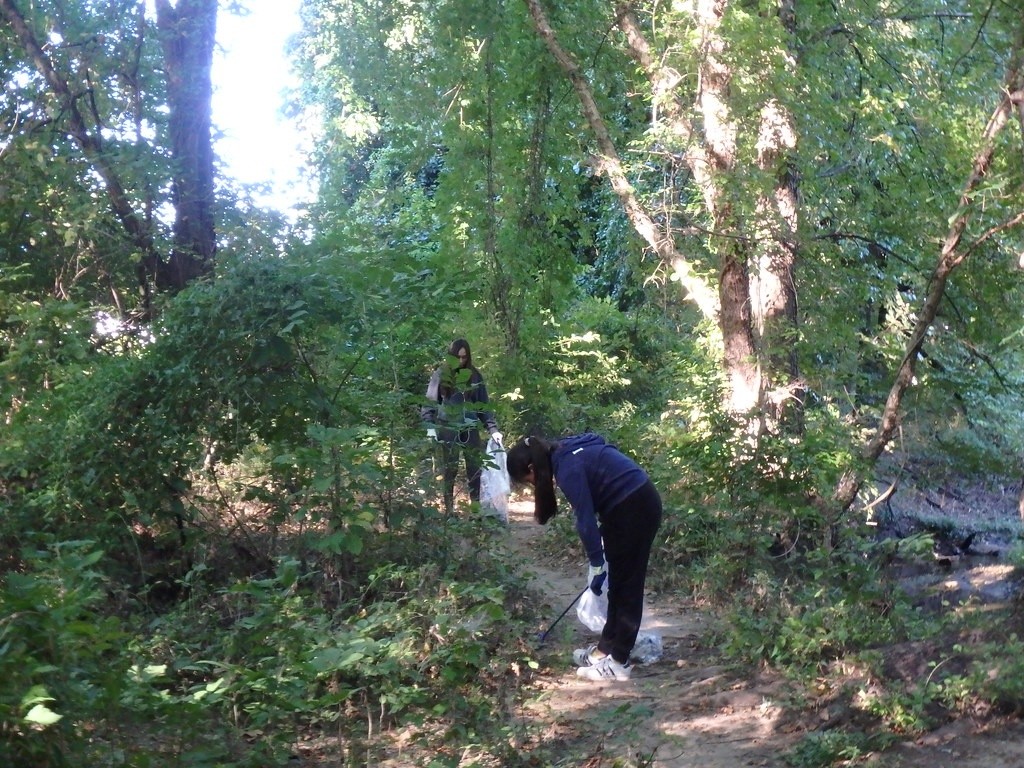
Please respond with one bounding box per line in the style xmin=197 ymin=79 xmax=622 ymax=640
xmin=426 ymin=428 xmax=438 ymax=443
xmin=590 ymin=570 xmax=607 ymax=596
xmin=492 ymin=431 xmax=503 ymax=445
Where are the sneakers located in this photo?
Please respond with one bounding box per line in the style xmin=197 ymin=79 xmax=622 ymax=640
xmin=572 ymin=645 xmax=601 ymax=667
xmin=576 ymin=654 xmax=632 ymax=681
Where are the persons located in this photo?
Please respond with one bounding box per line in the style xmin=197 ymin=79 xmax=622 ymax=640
xmin=420 ymin=338 xmax=503 ymax=522
xmin=506 ymin=433 xmax=662 ymax=681
